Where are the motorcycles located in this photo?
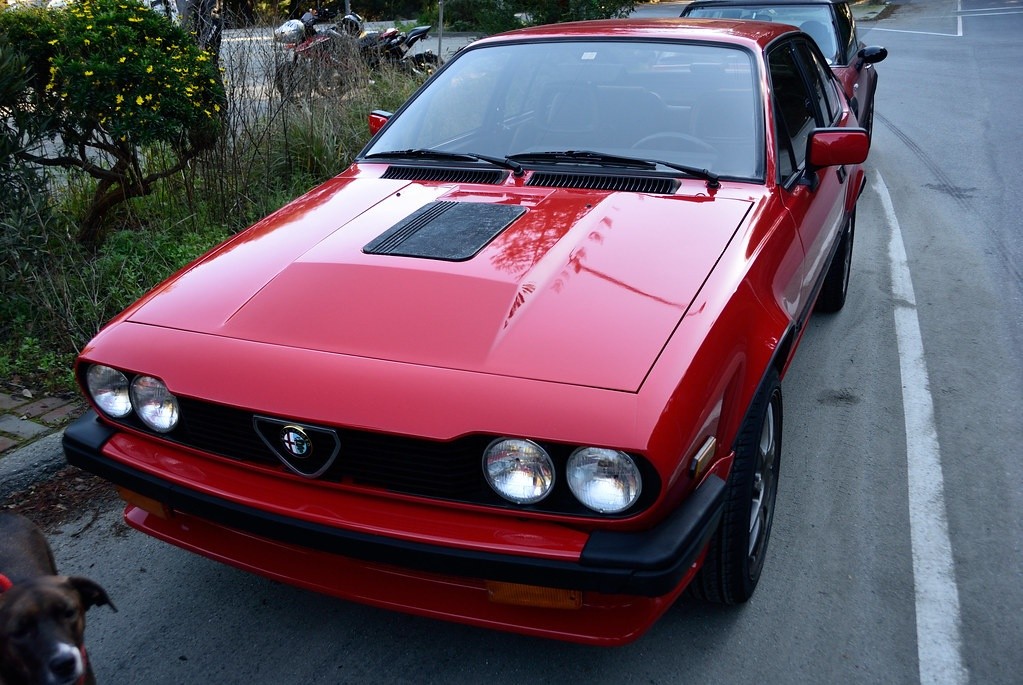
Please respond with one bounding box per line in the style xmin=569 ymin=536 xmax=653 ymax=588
xmin=272 ymin=9 xmax=448 ymax=101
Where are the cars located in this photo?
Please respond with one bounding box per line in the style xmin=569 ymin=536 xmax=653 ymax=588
xmin=608 ymin=1 xmax=888 ymax=149
xmin=66 ymin=18 xmax=876 ymax=647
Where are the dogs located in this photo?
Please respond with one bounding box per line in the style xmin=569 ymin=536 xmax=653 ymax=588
xmin=0 ymin=509 xmax=120 ymax=685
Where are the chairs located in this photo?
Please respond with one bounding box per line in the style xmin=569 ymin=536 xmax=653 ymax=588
xmin=798 ymin=19 xmax=835 ymax=57
xmin=506 ymin=89 xmax=614 ymax=152
xmin=688 ymin=86 xmax=753 ymax=174
